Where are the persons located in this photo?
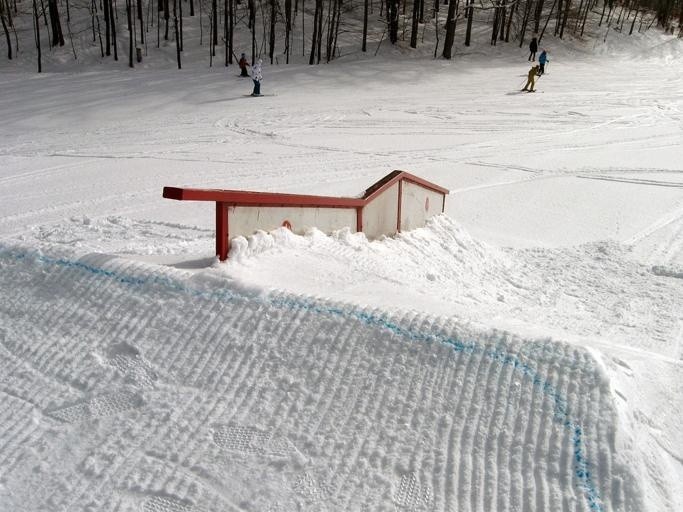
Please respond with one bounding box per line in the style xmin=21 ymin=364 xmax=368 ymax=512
xmin=524 ymin=64 xmax=539 ymax=90
xmin=539 ymin=51 xmax=549 ymax=73
xmin=250 ymin=59 xmax=263 ymax=94
xmin=529 ymin=38 xmax=537 ymax=61
xmin=239 ymin=52 xmax=250 ymax=76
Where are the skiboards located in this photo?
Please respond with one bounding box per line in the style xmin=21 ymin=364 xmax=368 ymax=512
xmin=517 ymin=88 xmax=543 ymax=93
xmin=242 ymin=93 xmax=276 ymax=98
